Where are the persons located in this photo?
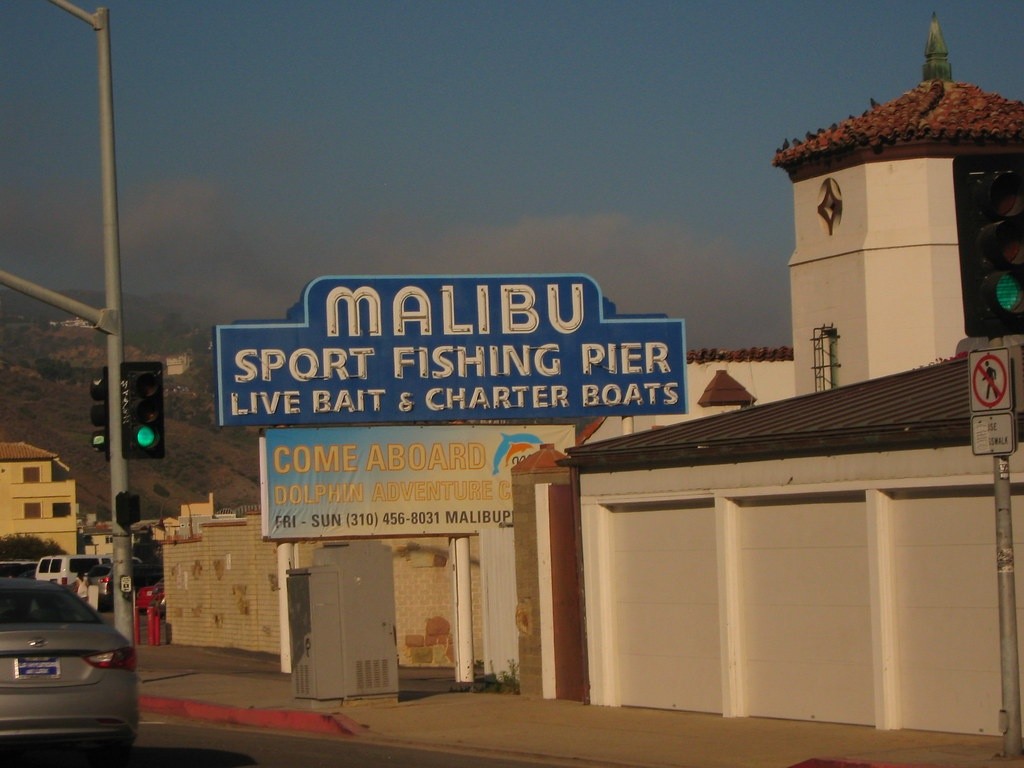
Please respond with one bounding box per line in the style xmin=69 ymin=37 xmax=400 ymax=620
xmin=75 ymin=570 xmax=89 ymax=598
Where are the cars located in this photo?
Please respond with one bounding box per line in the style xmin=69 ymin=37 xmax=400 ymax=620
xmin=0 ymin=577 xmax=140 ymax=768
xmin=0 ymin=550 xmax=166 ymax=622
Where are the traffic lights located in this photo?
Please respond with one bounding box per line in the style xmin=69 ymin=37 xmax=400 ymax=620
xmin=952 ymin=152 xmax=1024 ymax=339
xmin=120 ymin=361 xmax=166 ymax=461
xmin=89 ymin=364 xmax=110 ymax=463
